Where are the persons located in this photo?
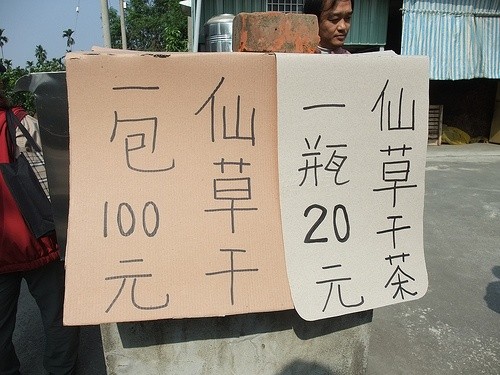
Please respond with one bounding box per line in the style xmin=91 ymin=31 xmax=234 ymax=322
xmin=0 ymin=62 xmax=81 ymax=375
xmin=303 ymin=0 xmax=354 ymax=53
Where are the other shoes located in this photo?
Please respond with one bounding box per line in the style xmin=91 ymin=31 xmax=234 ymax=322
xmin=47 ymin=359 xmax=77 ymax=375
xmin=0 ymin=370 xmax=21 ymax=375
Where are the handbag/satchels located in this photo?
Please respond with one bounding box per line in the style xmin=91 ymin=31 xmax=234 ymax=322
xmin=0 ymin=109 xmax=55 ymax=240
